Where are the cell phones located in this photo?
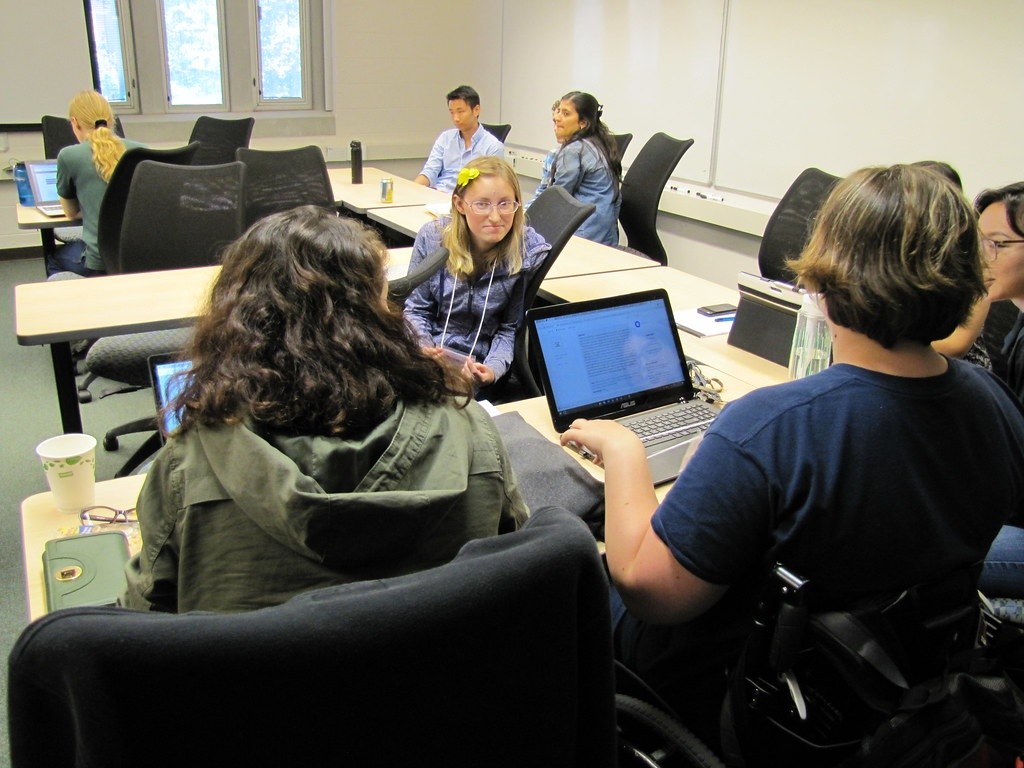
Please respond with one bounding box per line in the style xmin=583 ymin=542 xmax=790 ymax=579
xmin=697 ymin=304 xmax=738 ymax=317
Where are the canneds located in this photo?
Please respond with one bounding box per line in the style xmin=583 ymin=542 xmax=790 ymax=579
xmin=379 ymin=177 xmax=393 ymax=203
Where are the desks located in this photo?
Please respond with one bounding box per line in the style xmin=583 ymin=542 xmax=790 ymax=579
xmin=0 ymin=167 xmax=815 ymax=626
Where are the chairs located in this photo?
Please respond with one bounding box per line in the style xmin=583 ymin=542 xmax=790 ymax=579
xmin=8 ymin=506 xmax=1024 ymax=766
xmin=41 ymin=115 xmax=855 ymax=555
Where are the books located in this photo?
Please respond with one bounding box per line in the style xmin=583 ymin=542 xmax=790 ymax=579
xmin=674 ymin=310 xmax=736 ymax=339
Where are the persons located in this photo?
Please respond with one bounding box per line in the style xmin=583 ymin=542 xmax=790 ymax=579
xmin=414 ymin=85 xmax=506 ymax=193
xmin=403 ymin=157 xmax=552 ymax=400
xmin=46 ymin=92 xmax=143 ymax=277
xmin=114 ymin=203 xmax=532 ymax=615
xmin=911 ymin=162 xmax=1024 ymax=405
xmin=521 ymin=91 xmax=622 ymax=247
xmin=560 ymin=161 xmax=1024 ymax=767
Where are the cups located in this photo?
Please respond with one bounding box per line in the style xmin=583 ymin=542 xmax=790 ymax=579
xmin=36 ymin=434 xmax=96 ymax=513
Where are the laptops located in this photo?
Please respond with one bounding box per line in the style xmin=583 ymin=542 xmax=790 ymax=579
xmin=25 ymin=158 xmax=66 ymax=218
xmin=524 ymin=288 xmax=723 ymax=487
xmin=146 ymin=349 xmax=199 ymax=441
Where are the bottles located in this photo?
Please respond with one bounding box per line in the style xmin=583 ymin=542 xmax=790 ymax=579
xmin=14 ymin=163 xmax=35 ymax=207
xmin=786 ymin=292 xmax=831 ymax=382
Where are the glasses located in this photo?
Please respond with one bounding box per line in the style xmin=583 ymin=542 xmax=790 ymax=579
xmin=982 ymin=238 xmax=1024 ymax=262
xmin=462 ymin=199 xmax=522 ymax=216
xmin=79 ymin=506 xmax=140 ymax=527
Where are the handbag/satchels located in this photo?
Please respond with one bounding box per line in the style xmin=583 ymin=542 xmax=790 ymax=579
xmin=42 ymin=532 xmax=131 ymax=613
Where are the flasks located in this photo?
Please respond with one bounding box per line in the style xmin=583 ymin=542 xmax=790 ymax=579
xmin=351 ymin=140 xmax=362 ymax=185
